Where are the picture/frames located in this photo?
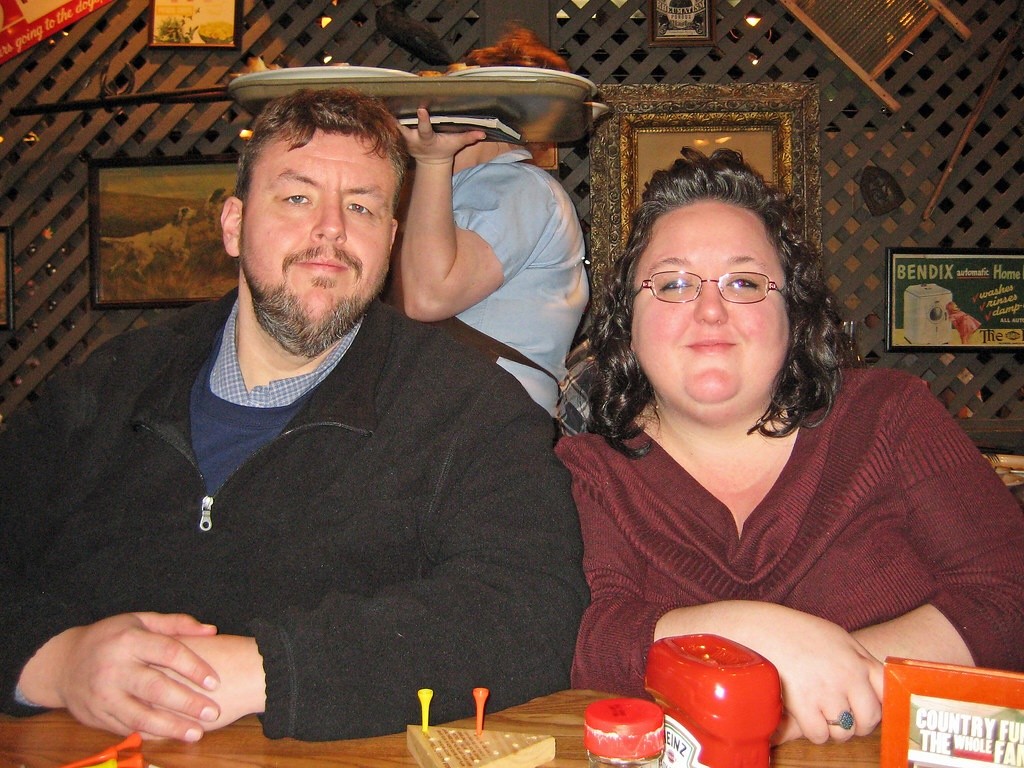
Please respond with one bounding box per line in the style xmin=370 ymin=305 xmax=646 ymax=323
xmin=647 ymin=0 xmax=718 ymax=48
xmin=146 ymin=0 xmax=244 ymax=50
xmin=884 ymin=246 xmax=1024 ymax=354
xmin=85 ymin=153 xmax=247 ymax=312
xmin=589 ymin=81 xmax=822 ymax=312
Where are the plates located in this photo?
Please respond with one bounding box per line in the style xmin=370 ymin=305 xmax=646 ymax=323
xmin=448 ymin=65 xmax=597 ymax=97
xmin=593 ymin=102 xmax=609 ymax=122
xmin=230 ymin=66 xmax=420 ymax=87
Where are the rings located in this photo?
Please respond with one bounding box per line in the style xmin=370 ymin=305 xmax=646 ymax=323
xmin=825 ymin=707 xmax=855 ymax=730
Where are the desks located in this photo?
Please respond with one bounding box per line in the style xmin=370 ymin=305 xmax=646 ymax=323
xmin=0 ymin=689 xmax=891 ymax=768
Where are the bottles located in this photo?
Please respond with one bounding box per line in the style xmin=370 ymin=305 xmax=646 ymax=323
xmin=584 ymin=698 xmax=665 ymax=768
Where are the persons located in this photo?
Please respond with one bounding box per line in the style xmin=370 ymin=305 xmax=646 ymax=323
xmin=1 ymin=85 xmax=594 ymax=743
xmin=392 ymin=107 xmax=596 ymax=444
xmin=551 ymin=145 xmax=1024 ymax=745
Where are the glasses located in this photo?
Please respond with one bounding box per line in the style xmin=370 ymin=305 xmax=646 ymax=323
xmin=633 ymin=271 xmax=786 ymax=304
